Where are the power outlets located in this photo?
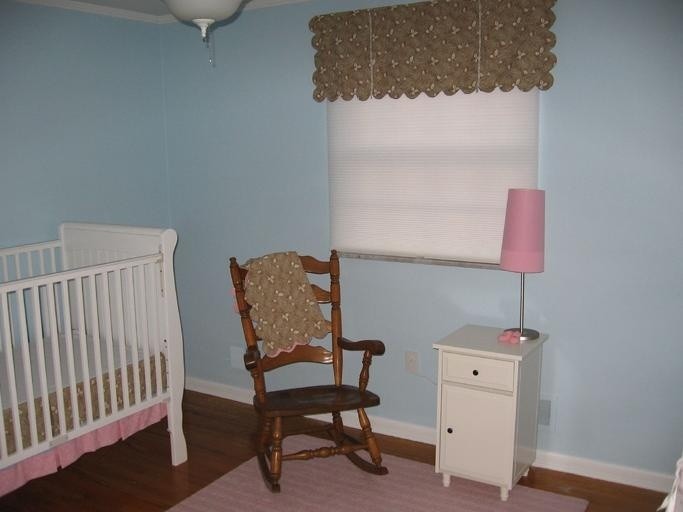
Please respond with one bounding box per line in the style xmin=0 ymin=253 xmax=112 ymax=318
xmin=404 ymin=351 xmax=418 ymax=374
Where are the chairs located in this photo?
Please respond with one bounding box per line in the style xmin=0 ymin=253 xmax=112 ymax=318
xmin=229 ymin=249 xmax=388 ymax=493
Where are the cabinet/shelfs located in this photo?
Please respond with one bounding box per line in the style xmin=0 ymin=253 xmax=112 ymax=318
xmin=431 ymin=323 xmax=549 ymax=502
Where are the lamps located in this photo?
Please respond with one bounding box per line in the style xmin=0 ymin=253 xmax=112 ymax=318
xmin=163 ymin=0 xmax=243 ymax=40
xmin=498 ymin=188 xmax=545 ymax=340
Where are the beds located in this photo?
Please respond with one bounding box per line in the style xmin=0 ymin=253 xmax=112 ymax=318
xmin=0 ymin=221 xmax=189 ymax=498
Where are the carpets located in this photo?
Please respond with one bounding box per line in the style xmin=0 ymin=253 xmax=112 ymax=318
xmin=164 ymin=433 xmax=590 ymax=512
